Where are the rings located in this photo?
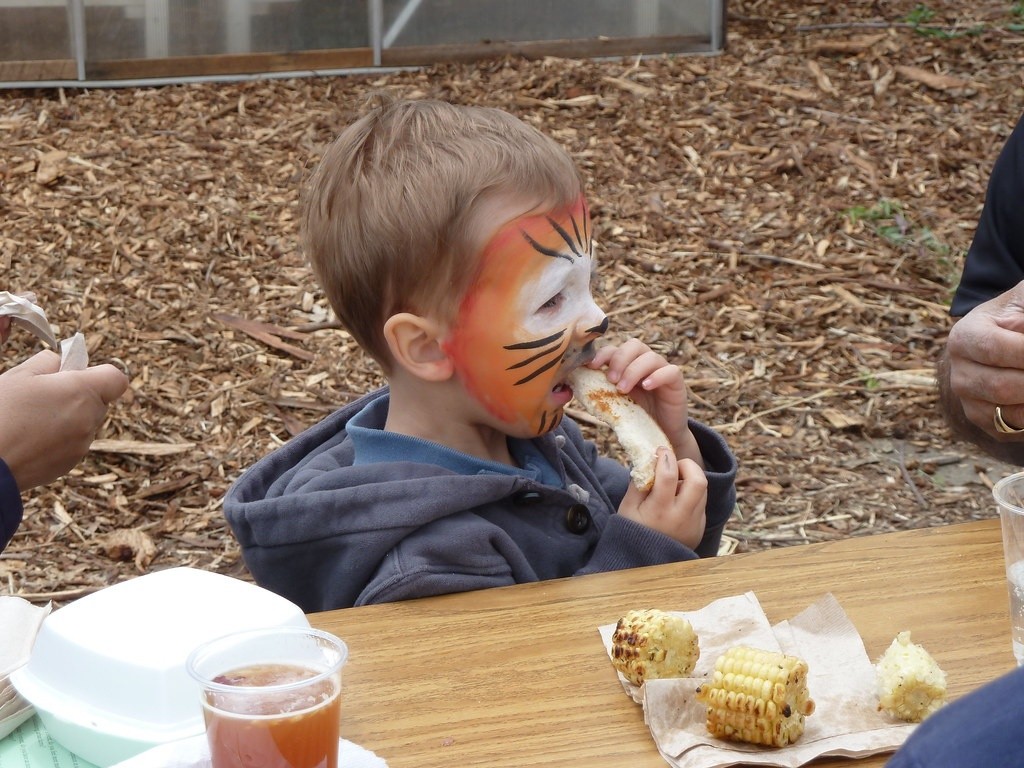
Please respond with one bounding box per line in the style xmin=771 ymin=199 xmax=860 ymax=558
xmin=992 ymin=402 xmax=1024 ymax=434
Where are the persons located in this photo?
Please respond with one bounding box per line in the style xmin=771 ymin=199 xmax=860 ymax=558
xmin=884 ymin=664 xmax=1024 ymax=768
xmin=942 ymin=114 xmax=1024 ymax=459
xmin=217 ymin=97 xmax=735 ymax=617
xmin=0 ymin=312 xmax=131 ymax=556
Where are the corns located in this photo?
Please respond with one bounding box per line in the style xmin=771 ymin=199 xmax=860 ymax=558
xmin=610 ymin=608 xmax=701 ymax=686
xmin=696 ymin=646 xmax=816 ymax=748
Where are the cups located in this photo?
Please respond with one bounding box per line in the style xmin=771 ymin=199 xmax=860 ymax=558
xmin=186 ymin=625 xmax=348 ymax=768
xmin=993 ymin=471 xmax=1024 ymax=667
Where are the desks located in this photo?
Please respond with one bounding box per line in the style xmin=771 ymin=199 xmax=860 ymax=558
xmin=309 ymin=514 xmax=1024 ymax=768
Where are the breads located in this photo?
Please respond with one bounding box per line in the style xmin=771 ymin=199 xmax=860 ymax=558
xmin=561 ymin=366 xmax=674 ymax=490
